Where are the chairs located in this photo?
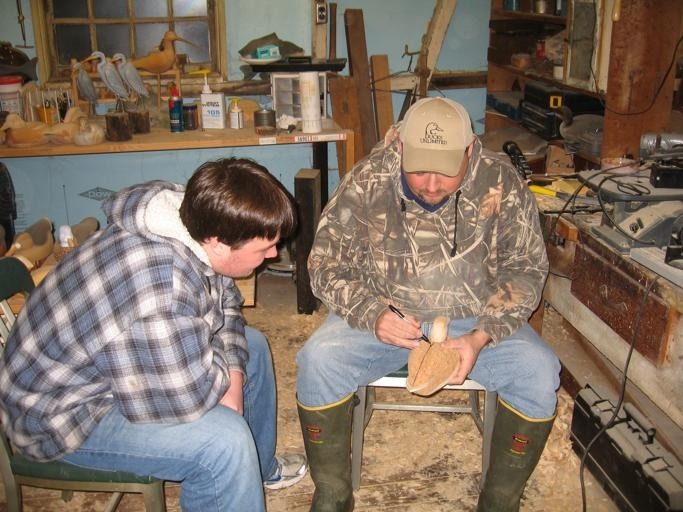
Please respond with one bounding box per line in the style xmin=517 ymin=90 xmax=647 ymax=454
xmin=1 ymin=255 xmax=173 ymax=511
xmin=351 ymin=255 xmax=498 ymax=496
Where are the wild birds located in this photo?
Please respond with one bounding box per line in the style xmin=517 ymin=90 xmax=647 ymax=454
xmin=109 ymin=53 xmax=150 ymax=111
xmin=70 ymin=62 xmax=98 ymax=115
xmin=83 ymin=51 xmax=130 ymax=113
xmin=131 ymin=30 xmax=200 ymax=110
xmin=546 ymin=106 xmax=605 ymax=141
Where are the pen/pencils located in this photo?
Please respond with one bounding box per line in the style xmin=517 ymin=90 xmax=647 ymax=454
xmin=389 ymin=305 xmax=431 ymax=345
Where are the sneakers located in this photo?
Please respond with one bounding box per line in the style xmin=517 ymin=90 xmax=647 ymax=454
xmin=263 ymin=453 xmax=309 ymax=489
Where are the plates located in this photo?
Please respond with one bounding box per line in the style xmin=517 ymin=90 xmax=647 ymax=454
xmin=239 ymin=53 xmax=282 ymax=66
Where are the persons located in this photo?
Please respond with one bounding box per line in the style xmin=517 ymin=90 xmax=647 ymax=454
xmin=0 ymin=155 xmax=311 ymax=512
xmin=291 ymin=96 xmax=561 ymax=512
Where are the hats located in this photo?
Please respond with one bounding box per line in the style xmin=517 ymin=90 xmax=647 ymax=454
xmin=398 ymin=97 xmax=475 ymax=178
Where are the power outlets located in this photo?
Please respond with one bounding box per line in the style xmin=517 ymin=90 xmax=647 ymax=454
xmin=316 ymin=3 xmax=327 ymax=24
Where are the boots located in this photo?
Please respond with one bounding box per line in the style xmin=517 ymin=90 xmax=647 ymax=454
xmin=476 ymin=397 xmax=559 ymax=511
xmin=294 ymin=391 xmax=358 ymax=511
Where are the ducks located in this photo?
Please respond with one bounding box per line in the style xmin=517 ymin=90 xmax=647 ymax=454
xmin=43 ymin=106 xmax=88 ymax=146
xmin=73 ymin=117 xmax=106 ymax=146
xmin=1 ymin=113 xmax=52 ymax=149
xmin=405 ymin=314 xmax=463 ymax=397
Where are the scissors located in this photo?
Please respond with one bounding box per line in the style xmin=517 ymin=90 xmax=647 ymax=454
xmin=604 ymin=175 xmax=650 ymax=196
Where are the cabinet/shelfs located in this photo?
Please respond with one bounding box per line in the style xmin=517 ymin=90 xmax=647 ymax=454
xmin=488 ymin=0 xmax=683 ymax=465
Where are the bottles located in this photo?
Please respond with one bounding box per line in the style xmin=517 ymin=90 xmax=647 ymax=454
xmin=182 ymin=103 xmax=198 ymax=130
xmin=169 ymin=100 xmax=183 ymax=132
xmin=168 ymin=87 xmax=180 ymax=111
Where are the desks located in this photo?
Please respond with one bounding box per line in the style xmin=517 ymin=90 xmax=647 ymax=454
xmin=0 ymin=107 xmax=356 ymax=173
